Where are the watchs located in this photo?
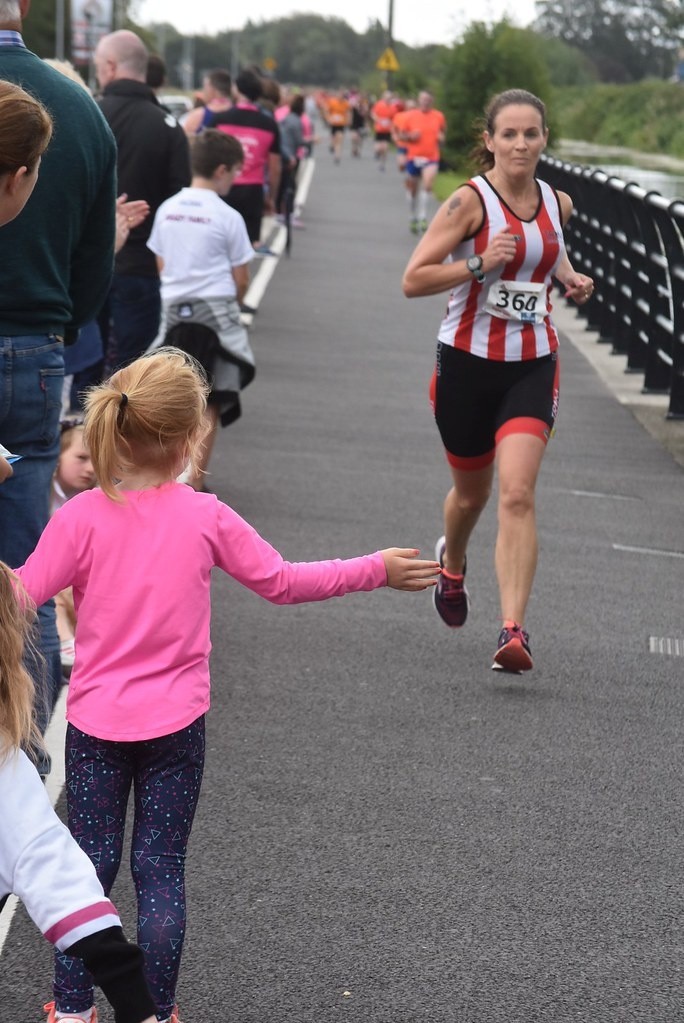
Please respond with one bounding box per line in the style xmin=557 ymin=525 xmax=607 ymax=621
xmin=466 ymin=255 xmax=486 ymax=283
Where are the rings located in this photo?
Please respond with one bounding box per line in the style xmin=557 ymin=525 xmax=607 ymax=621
xmin=585 ymin=290 xmax=588 ymax=293
xmin=585 ymin=294 xmax=588 ymax=298
xmin=129 ymin=217 xmax=132 ymax=220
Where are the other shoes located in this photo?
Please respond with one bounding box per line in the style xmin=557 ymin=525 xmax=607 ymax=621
xmin=421 ymin=219 xmax=427 ymax=230
xmin=59 ymin=638 xmax=77 ymax=665
xmin=410 ymin=220 xmax=421 ymax=233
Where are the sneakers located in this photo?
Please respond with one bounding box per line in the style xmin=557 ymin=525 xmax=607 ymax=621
xmin=489 ymin=621 xmax=534 ymax=675
xmin=432 ymin=535 xmax=472 ymax=628
xmin=44 ymin=1001 xmax=96 ymax=1023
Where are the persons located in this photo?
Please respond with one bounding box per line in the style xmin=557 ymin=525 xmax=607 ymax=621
xmin=147 ymin=130 xmax=253 ymax=496
xmin=95 ymin=30 xmax=192 ymax=367
xmin=187 ymin=64 xmax=323 ymax=256
xmin=399 ymin=91 xmax=445 ymax=234
xmin=318 ymin=85 xmax=420 ymax=201
xmin=0 ymin=346 xmax=442 ymax=1023
xmin=0 ymin=0 xmax=150 ymax=780
xmin=401 ymin=87 xmax=595 ymax=677
xmin=0 ymin=564 xmax=158 ymax=1023
